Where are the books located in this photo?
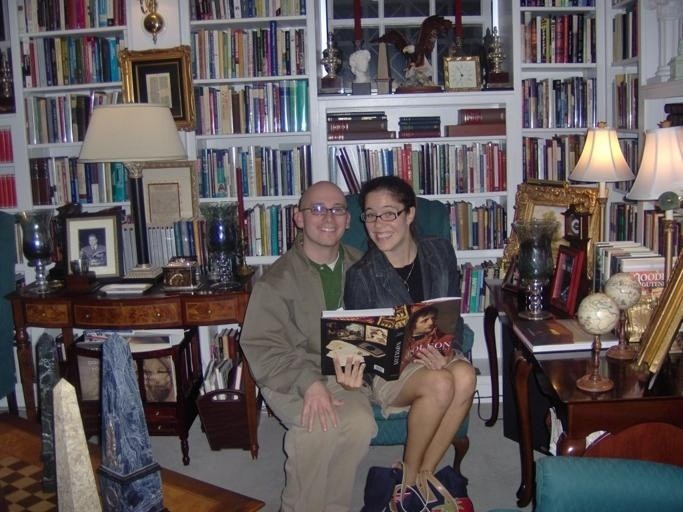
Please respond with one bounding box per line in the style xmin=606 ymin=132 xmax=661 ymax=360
xmin=200 ymin=328 xmax=258 ymax=401
xmin=1 ymin=1 xmax=130 ymax=209
xmin=447 ymin=199 xmax=510 ymax=314
xmin=320 ymin=296 xmax=461 ymax=381
xmin=189 ymin=0 xmax=313 ymax=256
xmin=327 ymin=108 xmax=506 ymax=195
xmin=521 ymin=0 xmax=683 ymax=341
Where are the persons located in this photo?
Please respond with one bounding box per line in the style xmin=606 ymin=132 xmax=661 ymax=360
xmin=400 ymin=305 xmax=453 ymax=371
xmin=343 ymin=176 xmax=476 ymax=512
xmin=238 ymin=181 xmax=379 ymax=512
xmin=80 ymin=233 xmax=107 ymax=266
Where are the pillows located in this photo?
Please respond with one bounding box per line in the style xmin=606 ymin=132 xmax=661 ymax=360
xmin=535 ymin=456 xmax=683 ymax=512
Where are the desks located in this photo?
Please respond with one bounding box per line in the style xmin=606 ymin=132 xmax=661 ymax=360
xmin=483 ymin=278 xmax=683 ymax=507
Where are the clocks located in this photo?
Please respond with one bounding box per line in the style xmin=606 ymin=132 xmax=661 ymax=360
xmin=443 ymin=54 xmax=483 ymax=93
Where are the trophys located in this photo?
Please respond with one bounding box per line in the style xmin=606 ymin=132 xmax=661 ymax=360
xmin=319 ymin=34 xmax=346 ymax=91
xmin=349 ymin=1 xmax=514 ymax=94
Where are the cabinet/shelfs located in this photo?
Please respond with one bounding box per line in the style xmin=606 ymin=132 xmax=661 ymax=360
xmin=609 ymin=0 xmax=643 ymax=246
xmin=4 ymin=265 xmax=261 ymax=459
xmin=68 ymin=326 xmax=203 ymax=465
xmin=0 ymin=0 xmax=133 ymax=408
xmin=180 ymin=0 xmax=605 ymax=405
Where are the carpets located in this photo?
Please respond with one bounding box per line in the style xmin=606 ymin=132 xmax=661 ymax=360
xmin=0 ymin=408 xmax=265 ymax=512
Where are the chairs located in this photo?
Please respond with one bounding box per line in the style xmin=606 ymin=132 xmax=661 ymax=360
xmin=342 ymin=193 xmax=474 ymax=474
xmin=557 ymin=423 xmax=683 ymax=512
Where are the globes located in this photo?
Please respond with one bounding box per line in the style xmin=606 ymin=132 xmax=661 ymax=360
xmin=575 ymin=292 xmax=621 ymax=393
xmin=604 ymin=271 xmax=642 ymax=361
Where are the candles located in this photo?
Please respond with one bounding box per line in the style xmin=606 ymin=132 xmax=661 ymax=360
xmin=236 ymin=168 xmax=243 ymax=228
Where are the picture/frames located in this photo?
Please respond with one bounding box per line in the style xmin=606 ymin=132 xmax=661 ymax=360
xmin=61 ymin=213 xmax=125 ymax=282
xmin=634 ymin=249 xmax=683 ymax=390
xmin=500 ymin=184 xmax=601 ymax=315
xmin=130 ymin=160 xmax=200 ymax=224
xmin=117 ymin=45 xmax=197 ymax=133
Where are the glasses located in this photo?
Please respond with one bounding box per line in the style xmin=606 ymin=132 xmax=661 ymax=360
xmin=298 ymin=203 xmax=347 ymax=217
xmin=360 ymin=208 xmax=407 ymax=223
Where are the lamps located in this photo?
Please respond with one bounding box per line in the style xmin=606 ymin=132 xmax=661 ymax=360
xmin=627 ymin=121 xmax=683 ymax=287
xmin=569 ymin=121 xmax=634 ymax=246
xmin=76 ymin=103 xmax=189 ymax=285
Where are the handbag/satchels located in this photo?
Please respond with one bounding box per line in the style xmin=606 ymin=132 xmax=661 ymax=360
xmin=358 ymin=462 xmax=479 ymax=512
